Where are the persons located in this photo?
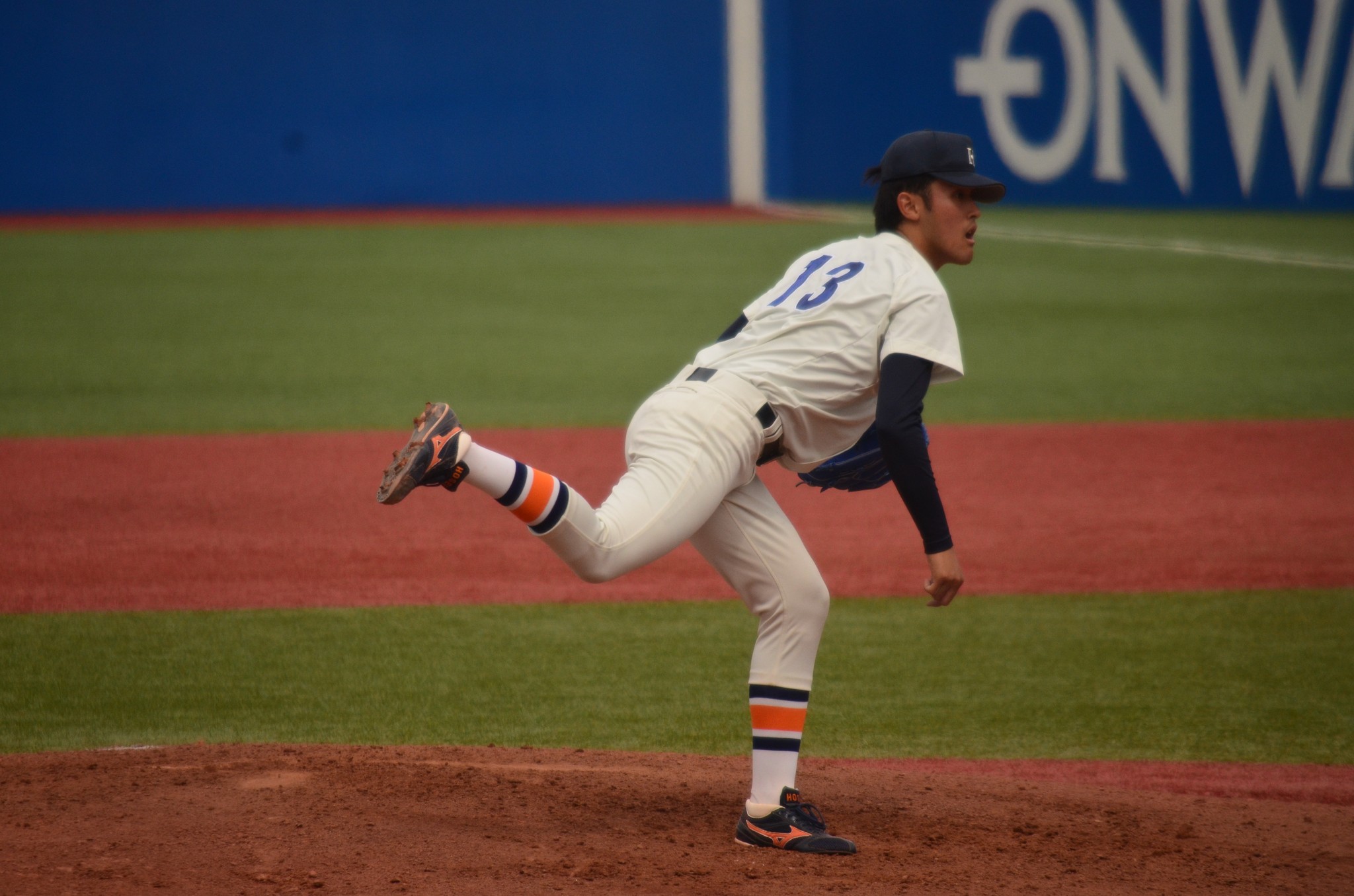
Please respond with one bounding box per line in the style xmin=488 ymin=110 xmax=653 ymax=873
xmin=380 ymin=133 xmax=1008 ymax=856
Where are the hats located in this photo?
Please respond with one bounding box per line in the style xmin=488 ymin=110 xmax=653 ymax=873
xmin=877 ymin=131 xmax=1006 ymax=203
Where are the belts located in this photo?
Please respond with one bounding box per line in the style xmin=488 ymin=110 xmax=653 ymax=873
xmin=689 ymin=369 xmax=785 ymax=466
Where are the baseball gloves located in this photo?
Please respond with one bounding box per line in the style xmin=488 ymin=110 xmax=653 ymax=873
xmin=797 ymin=419 xmax=929 ymax=493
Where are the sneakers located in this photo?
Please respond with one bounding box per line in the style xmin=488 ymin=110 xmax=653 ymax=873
xmin=378 ymin=400 xmax=474 ymax=506
xmin=735 ymin=785 xmax=857 ymax=858
